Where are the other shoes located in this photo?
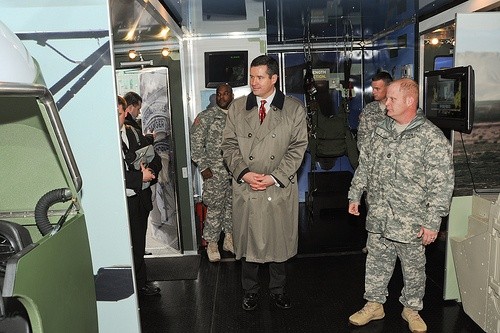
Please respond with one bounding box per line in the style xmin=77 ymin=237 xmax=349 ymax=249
xmin=144 ymin=251 xmax=152 ymax=255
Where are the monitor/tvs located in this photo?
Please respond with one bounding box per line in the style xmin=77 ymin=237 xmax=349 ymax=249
xmin=423 ymin=65 xmax=475 ymax=135
xmin=204 ymin=50 xmax=248 ymax=88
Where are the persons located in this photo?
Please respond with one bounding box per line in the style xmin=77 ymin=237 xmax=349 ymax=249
xmin=348 ymin=78 xmax=455 ymax=333
xmin=190 ymin=82 xmax=235 ymax=262
xmin=117 ymin=96 xmax=162 ymax=297
xmin=123 ymin=92 xmax=156 ymax=256
xmin=356 ymin=72 xmax=394 ymax=252
xmin=220 ymin=55 xmax=309 ymax=312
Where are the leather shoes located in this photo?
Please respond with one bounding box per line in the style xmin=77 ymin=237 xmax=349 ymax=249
xmin=242 ymin=292 xmax=258 ymax=310
xmin=270 ymin=292 xmax=291 ymax=308
xmin=137 ymin=282 xmax=162 ymax=296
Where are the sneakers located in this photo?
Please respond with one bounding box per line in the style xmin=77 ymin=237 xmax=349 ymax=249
xmin=207 ymin=243 xmax=221 ymax=262
xmin=401 ymin=307 xmax=427 ymax=333
xmin=222 ymin=237 xmax=235 ymax=255
xmin=348 ymin=301 xmax=385 ymax=326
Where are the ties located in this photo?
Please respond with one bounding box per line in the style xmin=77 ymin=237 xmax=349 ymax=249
xmin=120 ymin=131 xmax=129 ymax=155
xmin=258 ymin=100 xmax=267 ymax=124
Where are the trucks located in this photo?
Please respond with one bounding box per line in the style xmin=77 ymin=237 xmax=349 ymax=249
xmin=0 ymin=20 xmax=99 ymax=333
